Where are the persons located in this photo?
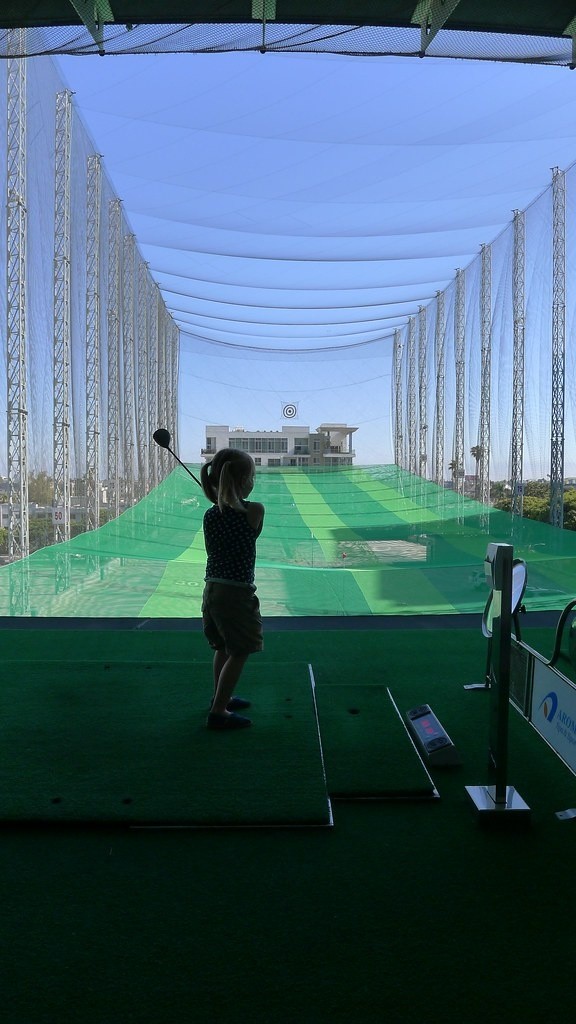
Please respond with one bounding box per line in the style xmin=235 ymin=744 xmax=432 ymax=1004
xmin=200 ymin=448 xmax=264 ymax=731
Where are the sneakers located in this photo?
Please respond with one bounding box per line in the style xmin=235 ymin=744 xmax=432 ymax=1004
xmin=207 ymin=711 xmax=252 ymax=728
xmin=210 ymin=695 xmax=251 ymax=709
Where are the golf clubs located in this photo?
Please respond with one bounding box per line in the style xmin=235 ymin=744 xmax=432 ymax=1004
xmin=153 ymin=429 xmax=203 ymax=487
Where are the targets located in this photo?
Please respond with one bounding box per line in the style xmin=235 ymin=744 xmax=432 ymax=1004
xmin=280 ymin=401 xmax=298 ymax=419
